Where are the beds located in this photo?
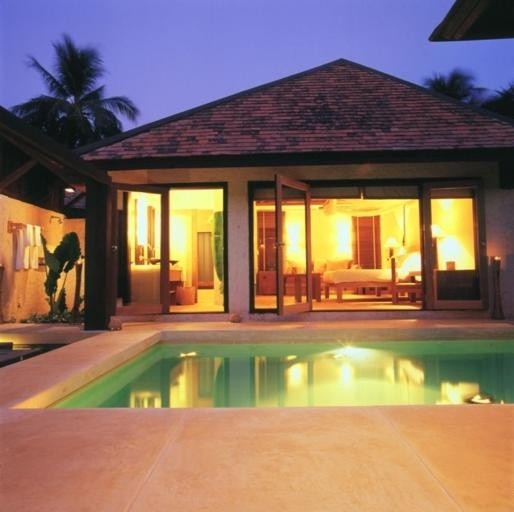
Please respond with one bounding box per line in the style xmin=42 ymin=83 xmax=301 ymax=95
xmin=322 ymin=269 xmax=405 ymax=303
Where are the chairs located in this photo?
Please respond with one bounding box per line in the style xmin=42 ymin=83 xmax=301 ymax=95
xmin=390 ymin=257 xmax=421 ymax=304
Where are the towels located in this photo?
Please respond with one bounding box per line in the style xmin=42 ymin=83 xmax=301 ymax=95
xmin=16 ymin=223 xmax=41 ymax=270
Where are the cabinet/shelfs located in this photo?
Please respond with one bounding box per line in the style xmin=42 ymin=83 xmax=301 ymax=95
xmin=256 ymin=271 xmax=321 ymax=295
